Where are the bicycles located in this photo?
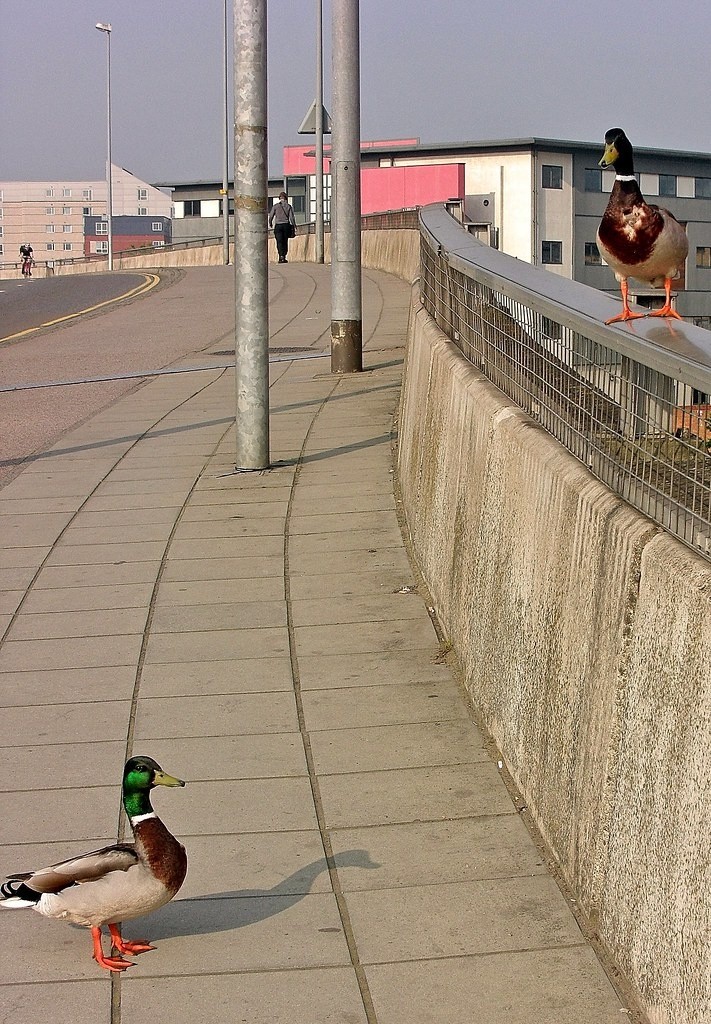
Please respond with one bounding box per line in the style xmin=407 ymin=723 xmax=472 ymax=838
xmin=22 ymin=256 xmax=32 ymax=279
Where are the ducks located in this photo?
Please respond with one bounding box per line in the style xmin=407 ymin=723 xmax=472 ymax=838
xmin=0 ymin=755 xmax=189 ymax=970
xmin=595 ymin=127 xmax=689 ymax=326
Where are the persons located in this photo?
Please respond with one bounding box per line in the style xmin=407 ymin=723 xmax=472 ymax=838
xmin=19 ymin=243 xmax=33 ymax=276
xmin=269 ymin=191 xmax=298 ymax=263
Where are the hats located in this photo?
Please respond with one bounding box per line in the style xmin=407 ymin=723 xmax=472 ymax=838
xmin=278 ymin=192 xmax=287 ymax=200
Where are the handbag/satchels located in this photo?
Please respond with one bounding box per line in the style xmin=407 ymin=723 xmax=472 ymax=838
xmin=287 ymin=224 xmax=295 ymax=238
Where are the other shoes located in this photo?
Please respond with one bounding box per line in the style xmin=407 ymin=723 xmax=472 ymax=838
xmin=278 ymin=256 xmax=288 ymax=263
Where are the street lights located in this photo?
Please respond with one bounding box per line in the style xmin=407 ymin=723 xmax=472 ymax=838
xmin=95 ymin=21 xmax=113 ymax=271
